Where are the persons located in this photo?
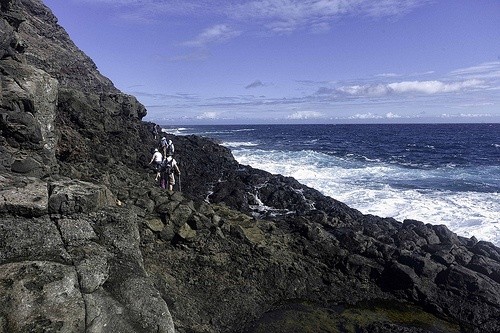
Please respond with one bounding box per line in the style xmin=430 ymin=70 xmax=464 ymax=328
xmin=149 ymin=147 xmax=163 ymax=181
xmin=162 ymin=151 xmax=180 ymax=191
xmin=166 ymin=139 xmax=174 ymax=159
xmin=159 ymin=136 xmax=169 ymax=160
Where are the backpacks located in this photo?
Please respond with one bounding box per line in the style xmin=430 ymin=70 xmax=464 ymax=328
xmin=167 ymin=144 xmax=172 ymax=152
xmin=165 ymin=158 xmax=173 ymax=174
xmin=161 ymin=141 xmax=166 ymax=147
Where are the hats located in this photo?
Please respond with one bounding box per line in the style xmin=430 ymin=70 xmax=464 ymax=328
xmin=167 ymin=152 xmax=174 ymax=156
xmin=161 ymin=137 xmax=166 ymax=140
xmin=168 ymin=140 xmax=172 ymax=144
xmin=155 ymin=148 xmax=158 ymax=151
